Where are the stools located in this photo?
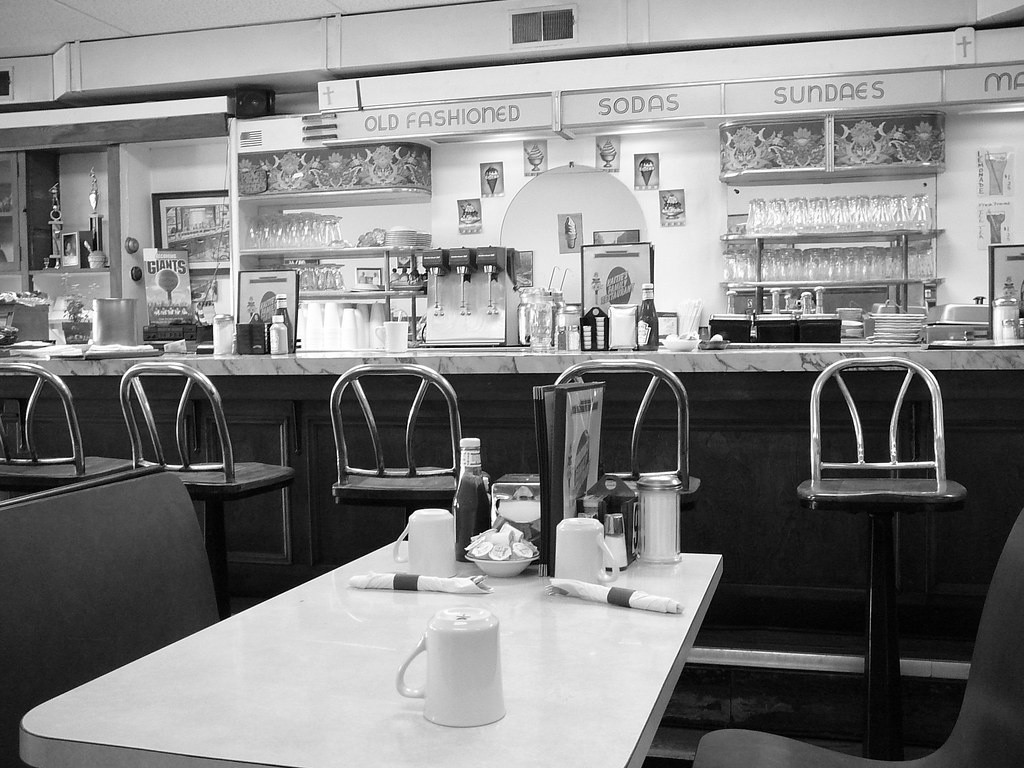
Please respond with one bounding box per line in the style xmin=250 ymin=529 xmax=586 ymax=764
xmin=0 ymin=362 xmax=134 ymax=499
xmin=329 ymin=361 xmax=462 ymax=540
xmin=119 ymin=359 xmax=296 ymax=620
xmin=796 ymin=357 xmax=969 ymax=762
xmin=554 ymin=359 xmax=702 ymax=512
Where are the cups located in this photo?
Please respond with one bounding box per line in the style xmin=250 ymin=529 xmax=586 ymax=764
xmin=396 ymin=606 xmax=506 ymax=727
xmin=237 ymin=322 xmax=265 ymax=354
xmin=164 ymin=338 xmax=187 ymax=354
xmin=245 ymin=210 xmax=345 ymax=249
xmin=254 ymin=264 xmax=346 ymax=291
xmin=744 ymin=195 xmax=935 ymax=231
xmin=393 ymin=508 xmax=457 ymax=578
xmin=295 ymin=299 xmax=408 ymax=353
xmin=555 ymin=518 xmax=619 ymax=585
xmin=721 ymin=241 xmax=936 ymax=281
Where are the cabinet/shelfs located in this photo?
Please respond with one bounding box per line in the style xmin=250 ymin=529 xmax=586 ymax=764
xmin=237 ymin=187 xmax=431 ymax=341
xmin=718 ymin=164 xmax=946 ymax=315
xmin=0 ymin=152 xmax=23 ymax=275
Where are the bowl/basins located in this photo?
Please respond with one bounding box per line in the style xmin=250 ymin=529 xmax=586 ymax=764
xmin=131 ymin=266 xmax=142 ymax=281
xmin=465 ymin=554 xmax=540 ymax=577
xmin=659 ymin=339 xmax=701 ymax=352
xmin=125 ymin=237 xmax=139 ymax=254
xmin=699 ymin=340 xmax=731 ymax=350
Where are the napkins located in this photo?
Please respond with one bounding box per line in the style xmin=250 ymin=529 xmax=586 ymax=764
xmin=348 ymin=572 xmax=495 ymax=593
xmin=548 ymin=576 xmax=685 ymax=615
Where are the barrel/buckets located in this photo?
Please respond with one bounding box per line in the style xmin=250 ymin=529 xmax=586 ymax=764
xmin=92 ymin=298 xmax=138 ymax=347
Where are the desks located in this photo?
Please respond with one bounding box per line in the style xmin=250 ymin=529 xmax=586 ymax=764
xmin=19 ymin=540 xmax=724 ymax=768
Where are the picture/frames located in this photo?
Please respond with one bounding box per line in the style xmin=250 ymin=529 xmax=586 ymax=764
xmin=151 ymin=189 xmax=230 ymax=276
xmin=355 ymin=267 xmax=383 ymax=285
xmin=987 ymin=244 xmax=1024 ymax=339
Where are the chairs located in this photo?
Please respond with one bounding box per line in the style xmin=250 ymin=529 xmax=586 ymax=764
xmin=692 ymin=508 xmax=1024 ymax=768
xmin=0 ymin=473 xmax=221 ymax=768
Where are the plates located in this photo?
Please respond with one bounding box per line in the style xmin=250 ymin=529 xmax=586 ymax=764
xmin=384 ymin=227 xmax=432 ymax=247
xmin=836 ymin=307 xmax=928 ymax=344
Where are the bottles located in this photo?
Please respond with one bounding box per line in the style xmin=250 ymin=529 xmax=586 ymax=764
xmin=452 ymin=437 xmax=491 ymax=562
xmin=636 ymin=475 xmax=682 ymax=569
xmin=213 ymin=314 xmax=234 ymax=355
xmin=518 ymin=288 xmax=581 ymax=351
xmin=992 ymin=296 xmax=1024 ymax=344
xmin=604 ymin=513 xmax=628 ymax=572
xmin=270 ymin=294 xmax=294 ymax=354
xmin=637 ymin=283 xmax=659 ymax=350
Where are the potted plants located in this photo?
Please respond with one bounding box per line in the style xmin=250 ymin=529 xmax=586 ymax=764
xmin=59 ymin=271 xmax=100 ymax=345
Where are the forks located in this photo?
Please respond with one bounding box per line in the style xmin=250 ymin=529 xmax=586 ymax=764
xmin=544 ymin=585 xmax=569 ymax=596
